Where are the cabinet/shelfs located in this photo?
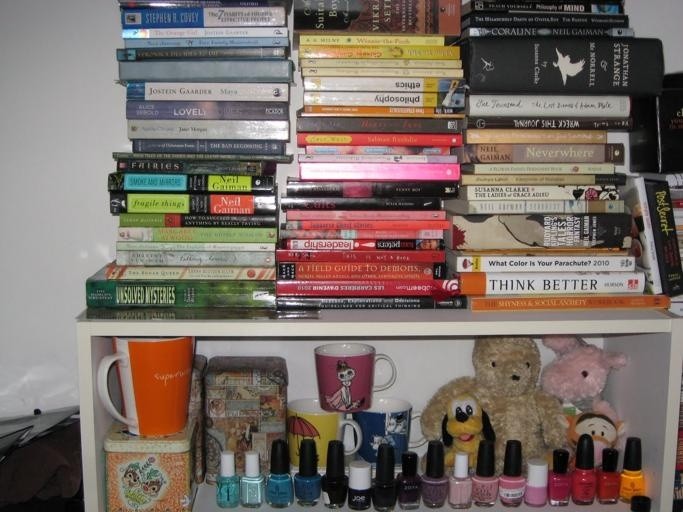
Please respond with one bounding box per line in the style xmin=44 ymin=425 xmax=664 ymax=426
xmin=74 ymin=308 xmax=683 ymax=512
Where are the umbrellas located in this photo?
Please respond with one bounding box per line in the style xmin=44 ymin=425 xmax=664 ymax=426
xmin=286 ymin=413 xmax=320 ymax=456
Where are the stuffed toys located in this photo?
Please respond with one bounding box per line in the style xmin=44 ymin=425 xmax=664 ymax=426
xmin=420 ymin=334 xmax=631 ymax=476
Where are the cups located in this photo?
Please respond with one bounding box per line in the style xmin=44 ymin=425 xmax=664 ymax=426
xmin=288 ymin=341 xmax=428 ymax=471
xmin=96 ymin=335 xmax=194 ymax=438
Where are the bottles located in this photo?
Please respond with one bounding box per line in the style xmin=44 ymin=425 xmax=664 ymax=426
xmin=396 ymin=452 xmax=423 ymax=509
xmin=422 ymin=440 xmax=449 ymax=509
xmin=372 ymin=442 xmax=398 ymax=511
xmin=499 ymin=439 xmax=526 ymax=506
xmin=346 ymin=462 xmax=372 ymax=510
xmin=217 ymin=450 xmax=239 ymax=507
xmin=597 ymin=447 xmax=621 ymax=504
xmin=321 ymin=440 xmax=349 ymax=510
xmin=572 ymin=435 xmax=597 ymax=506
xmin=264 ymin=438 xmax=294 ymax=508
xmin=449 ymin=451 xmax=473 ymax=509
xmin=620 ymin=437 xmax=646 ymax=503
xmin=526 ymin=458 xmax=549 ymax=506
xmin=548 ymin=449 xmax=572 ymax=506
xmin=472 ymin=439 xmax=498 ymax=505
xmin=241 ymin=452 xmax=265 ymax=508
xmin=293 ymin=439 xmax=322 ymax=507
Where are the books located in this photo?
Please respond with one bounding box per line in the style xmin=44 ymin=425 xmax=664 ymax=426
xmin=88 ymin=1 xmax=683 ymax=313
